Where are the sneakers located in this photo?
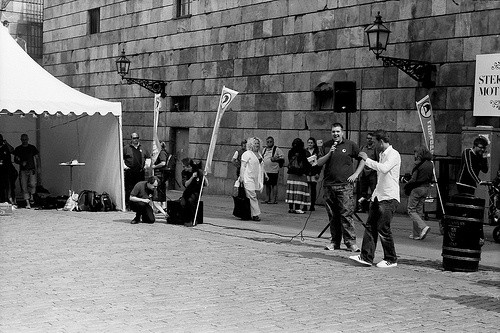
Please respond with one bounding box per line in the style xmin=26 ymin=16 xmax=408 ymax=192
xmin=409 ymin=234 xmax=420 ymax=240
xmin=324 ymin=242 xmax=340 ymax=250
xmin=347 ymin=244 xmax=360 ymax=252
xmin=420 ymin=226 xmax=431 ymax=240
xmin=376 ymin=260 xmax=397 ymax=268
xmin=349 ymin=255 xmax=372 ymax=266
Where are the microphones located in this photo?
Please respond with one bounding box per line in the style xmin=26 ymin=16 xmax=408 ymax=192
xmin=332 ymin=141 xmax=338 ymax=153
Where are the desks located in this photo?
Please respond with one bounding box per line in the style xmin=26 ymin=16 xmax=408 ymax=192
xmin=59 ymin=163 xmax=86 ymax=190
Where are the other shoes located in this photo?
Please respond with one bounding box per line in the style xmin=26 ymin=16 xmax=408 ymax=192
xmin=294 ymin=209 xmax=304 ymax=214
xmin=289 ymin=210 xmax=294 ymax=213
xmin=309 ymin=208 xmax=315 ymax=211
xmin=302 ymin=208 xmax=307 ymax=211
xmin=253 ymin=216 xmax=261 ymax=221
xmin=132 ymin=217 xmax=139 ymax=224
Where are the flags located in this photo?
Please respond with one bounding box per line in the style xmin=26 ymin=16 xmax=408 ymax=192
xmin=416 ymin=94 xmax=437 ymax=167
xmin=205 ymin=85 xmax=239 ymax=174
xmin=149 ymin=94 xmax=162 ymax=164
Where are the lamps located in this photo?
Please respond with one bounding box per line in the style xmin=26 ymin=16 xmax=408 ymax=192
xmin=362 ymin=11 xmax=437 ymax=89
xmin=115 ymin=49 xmax=167 ymax=99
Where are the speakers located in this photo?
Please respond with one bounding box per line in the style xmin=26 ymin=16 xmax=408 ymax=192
xmin=333 ymin=80 xmax=357 ymax=113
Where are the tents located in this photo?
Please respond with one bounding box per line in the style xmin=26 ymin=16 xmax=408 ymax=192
xmin=0 ymin=21 xmax=125 ymax=213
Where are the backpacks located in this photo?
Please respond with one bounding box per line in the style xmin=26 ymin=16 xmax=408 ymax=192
xmin=290 ymin=153 xmax=303 ymax=168
xmin=64 ymin=190 xmax=115 ymax=211
xmin=262 ymin=146 xmax=285 ymax=168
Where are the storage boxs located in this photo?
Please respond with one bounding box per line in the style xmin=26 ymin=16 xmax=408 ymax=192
xmin=0 ymin=203 xmax=13 ymax=216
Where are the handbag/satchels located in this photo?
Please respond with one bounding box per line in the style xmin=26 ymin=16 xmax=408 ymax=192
xmin=233 ymin=183 xmax=251 ymax=220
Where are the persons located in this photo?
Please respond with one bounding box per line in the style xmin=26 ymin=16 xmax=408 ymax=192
xmin=348 ymin=129 xmax=400 ymax=267
xmin=14 ymin=134 xmax=41 ymax=208
xmin=402 ymin=136 xmax=490 ymax=239
xmin=177 ymin=157 xmax=208 ymax=227
xmin=0 ymin=133 xmax=19 ymax=207
xmin=151 ymin=139 xmax=169 ymax=213
xmin=231 ymin=135 xmax=322 ymax=222
xmin=317 ymin=123 xmax=366 ymax=252
xmin=354 ymin=132 xmax=379 ymax=212
xmin=128 ymin=176 xmax=169 ymax=223
xmin=123 ymin=133 xmax=145 ymax=211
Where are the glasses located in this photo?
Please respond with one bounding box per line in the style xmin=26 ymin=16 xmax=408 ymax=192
xmin=132 ymin=137 xmax=139 ymax=139
xmin=477 ymin=146 xmax=487 ymax=149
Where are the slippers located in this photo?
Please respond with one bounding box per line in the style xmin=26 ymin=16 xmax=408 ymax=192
xmin=26 ymin=206 xmax=31 ymax=209
xmin=30 ymin=201 xmax=34 ymax=204
